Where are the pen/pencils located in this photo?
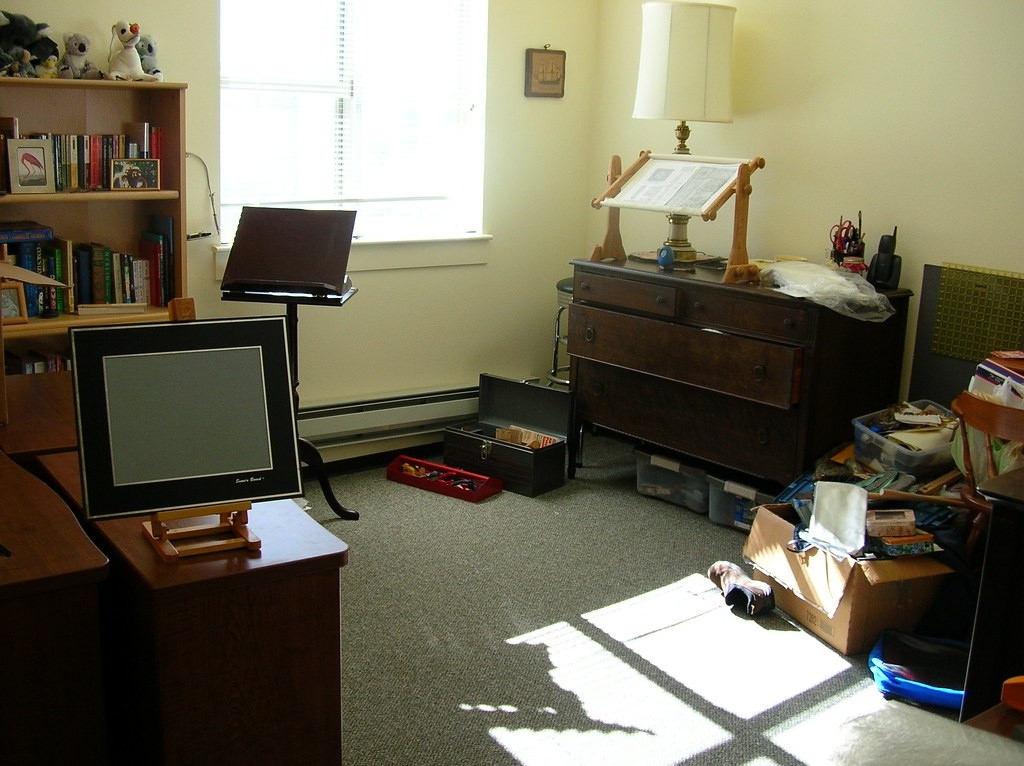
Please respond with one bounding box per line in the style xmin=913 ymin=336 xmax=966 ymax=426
xmin=837 ymin=211 xmax=865 ymax=257
xmin=659 ymin=266 xmax=696 ymax=273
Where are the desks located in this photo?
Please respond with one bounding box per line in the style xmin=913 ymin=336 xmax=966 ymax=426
xmin=957 ymin=468 xmax=1024 ymax=724
xmin=0 ymin=365 xmax=349 ymax=766
xmin=217 ymin=286 xmax=368 ymax=522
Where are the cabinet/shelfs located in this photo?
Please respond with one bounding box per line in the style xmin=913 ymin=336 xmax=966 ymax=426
xmin=0 ymin=71 xmax=189 ymax=340
xmin=558 ymin=255 xmax=917 ymax=526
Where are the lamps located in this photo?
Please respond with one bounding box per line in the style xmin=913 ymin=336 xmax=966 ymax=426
xmin=637 ymin=3 xmax=738 ymax=265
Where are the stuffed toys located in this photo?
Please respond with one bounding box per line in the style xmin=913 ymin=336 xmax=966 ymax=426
xmin=135 ymin=36 xmax=163 ymax=81
xmin=0 ymin=47 xmax=58 ymax=78
xmin=107 ymin=21 xmax=157 ymax=81
xmin=57 ymin=32 xmax=104 ymax=79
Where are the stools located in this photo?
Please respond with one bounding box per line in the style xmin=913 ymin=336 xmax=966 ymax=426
xmin=545 ymin=276 xmax=577 ymax=387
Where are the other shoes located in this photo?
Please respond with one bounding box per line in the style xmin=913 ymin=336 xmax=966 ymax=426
xmin=708 ymin=560 xmax=776 ymax=616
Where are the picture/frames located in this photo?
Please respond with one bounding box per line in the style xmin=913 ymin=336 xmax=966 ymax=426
xmin=523 ymin=46 xmax=566 ymax=98
xmin=8 ymin=139 xmax=57 ymax=194
xmin=111 ymin=158 xmax=160 ymax=191
xmin=1 ymin=282 xmax=29 ymax=324
xmin=69 ymin=317 xmax=304 ymax=521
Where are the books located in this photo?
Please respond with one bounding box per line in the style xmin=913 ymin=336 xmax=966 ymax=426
xmin=0 ymin=117 xmax=165 ymax=190
xmin=0 ymin=216 xmax=175 ymax=318
xmin=4 ymin=348 xmax=72 ymax=375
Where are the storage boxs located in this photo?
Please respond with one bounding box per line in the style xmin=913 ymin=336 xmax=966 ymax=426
xmin=440 ymin=374 xmax=577 ymax=497
xmin=632 ymin=441 xmax=712 ymax=517
xmin=741 ymin=497 xmax=956 ymax=658
xmin=707 ymin=470 xmax=791 ymax=533
xmin=850 ymin=399 xmax=959 ymax=479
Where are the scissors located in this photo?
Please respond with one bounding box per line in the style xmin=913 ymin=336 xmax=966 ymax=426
xmin=840 ymin=225 xmax=855 ymax=241
xmin=830 ymin=220 xmax=851 ymax=243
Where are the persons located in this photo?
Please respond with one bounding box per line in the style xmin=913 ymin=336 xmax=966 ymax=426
xmin=113 ymin=165 xmax=148 ymax=188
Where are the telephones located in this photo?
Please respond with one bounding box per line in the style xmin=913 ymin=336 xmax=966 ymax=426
xmin=866 ymin=226 xmax=902 ymax=291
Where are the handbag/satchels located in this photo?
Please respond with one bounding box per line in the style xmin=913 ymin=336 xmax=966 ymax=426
xmin=867 ymin=628 xmax=993 ymax=716
